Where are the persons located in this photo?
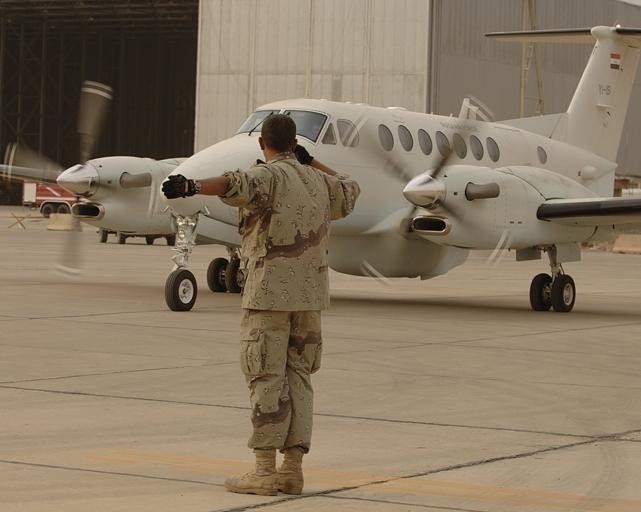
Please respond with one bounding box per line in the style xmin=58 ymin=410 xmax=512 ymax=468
xmin=162 ymin=114 xmax=360 ymax=498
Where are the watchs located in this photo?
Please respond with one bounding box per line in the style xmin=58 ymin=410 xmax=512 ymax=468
xmin=193 ymin=179 xmax=201 ymax=195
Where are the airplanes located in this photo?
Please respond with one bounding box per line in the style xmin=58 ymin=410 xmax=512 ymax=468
xmin=0 ymin=23 xmax=641 ymax=312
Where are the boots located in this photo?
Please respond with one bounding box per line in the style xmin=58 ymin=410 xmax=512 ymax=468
xmin=276 ymin=446 xmax=305 ymax=493
xmin=226 ymin=447 xmax=280 ymax=498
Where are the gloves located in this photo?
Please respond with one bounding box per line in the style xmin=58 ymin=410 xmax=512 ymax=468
xmin=160 ymin=173 xmax=199 ymax=199
xmin=294 ymin=145 xmax=313 ymax=163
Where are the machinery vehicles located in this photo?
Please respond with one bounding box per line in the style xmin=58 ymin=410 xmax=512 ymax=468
xmin=17 ymin=179 xmax=91 ymax=219
